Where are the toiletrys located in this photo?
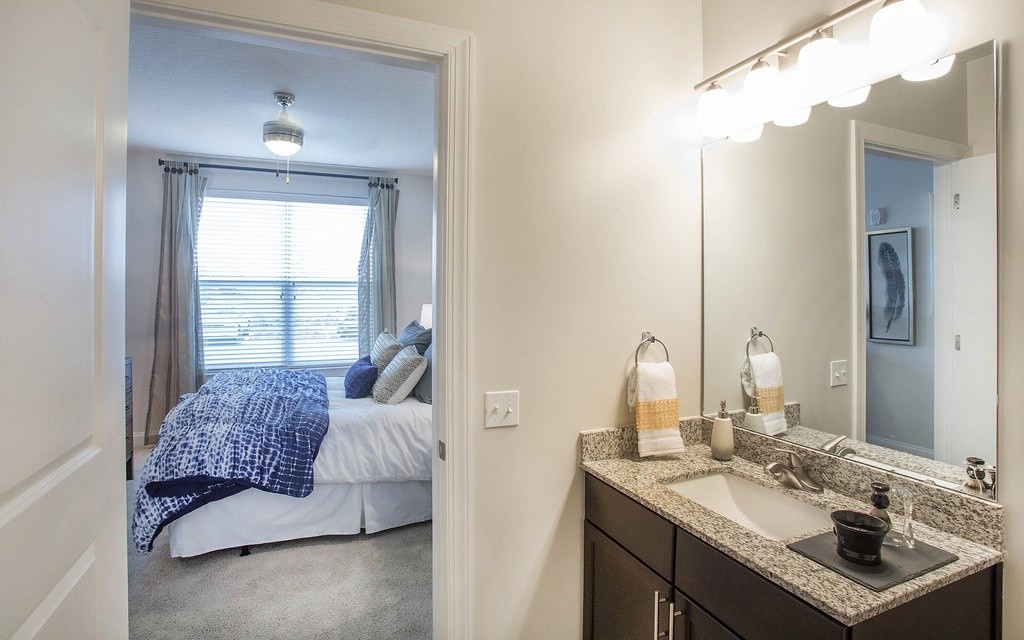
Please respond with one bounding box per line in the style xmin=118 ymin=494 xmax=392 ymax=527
xmin=711 ymin=401 xmax=735 ymax=460
xmin=743 ymin=396 xmax=766 ymax=433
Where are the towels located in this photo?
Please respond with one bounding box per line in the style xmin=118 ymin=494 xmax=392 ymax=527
xmin=740 ymin=351 xmax=787 ymax=436
xmin=627 ymin=361 xmax=685 ymax=459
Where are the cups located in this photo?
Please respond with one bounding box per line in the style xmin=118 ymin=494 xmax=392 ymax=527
xmin=830 ymin=510 xmax=890 ymax=566
xmin=982 ymin=465 xmax=997 ymax=499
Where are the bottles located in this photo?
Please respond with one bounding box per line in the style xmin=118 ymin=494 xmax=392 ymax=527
xmin=965 ymin=456 xmax=986 ymax=492
xmin=869 ymin=482 xmax=893 ymax=545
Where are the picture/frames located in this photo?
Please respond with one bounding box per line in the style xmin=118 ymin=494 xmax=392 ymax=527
xmin=865 ymin=227 xmax=916 ymax=346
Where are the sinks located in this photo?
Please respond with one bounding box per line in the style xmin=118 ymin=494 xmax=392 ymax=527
xmin=656 ymin=466 xmax=838 ymax=541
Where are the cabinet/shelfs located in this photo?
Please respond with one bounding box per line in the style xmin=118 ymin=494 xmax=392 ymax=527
xmin=126 ymin=357 xmax=134 ymax=480
xmin=584 ymin=470 xmax=1002 ymax=640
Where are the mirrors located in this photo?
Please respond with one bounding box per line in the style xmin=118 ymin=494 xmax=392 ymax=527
xmin=701 ymin=39 xmax=1001 ymax=503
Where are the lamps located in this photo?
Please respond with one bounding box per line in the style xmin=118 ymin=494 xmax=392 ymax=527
xmin=263 ymin=92 xmax=304 ymax=184
xmin=696 ymin=0 xmax=942 ymax=140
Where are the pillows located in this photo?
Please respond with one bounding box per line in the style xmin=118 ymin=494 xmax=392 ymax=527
xmin=344 ymin=319 xmax=432 ymax=404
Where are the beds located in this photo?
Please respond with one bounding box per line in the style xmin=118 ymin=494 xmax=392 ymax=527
xmin=132 ymin=305 xmax=433 ymax=559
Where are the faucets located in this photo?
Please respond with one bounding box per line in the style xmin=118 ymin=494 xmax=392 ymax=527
xmin=819 ymin=435 xmax=857 ymax=458
xmin=766 ymin=446 xmax=825 ymax=494
